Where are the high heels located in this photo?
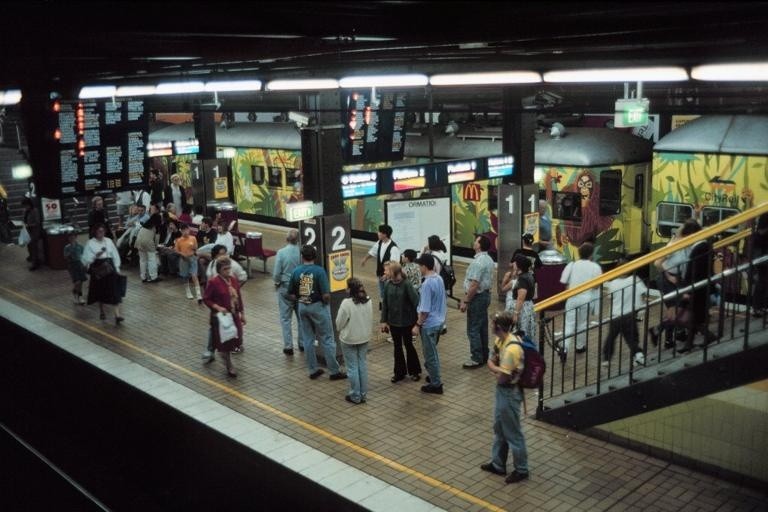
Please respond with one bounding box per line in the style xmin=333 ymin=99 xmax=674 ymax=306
xmin=115 ymin=316 xmax=125 ymax=324
xmin=99 ymin=314 xmax=106 ymax=321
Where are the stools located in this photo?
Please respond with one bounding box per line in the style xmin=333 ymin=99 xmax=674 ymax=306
xmin=245 ymin=238 xmax=277 ymax=274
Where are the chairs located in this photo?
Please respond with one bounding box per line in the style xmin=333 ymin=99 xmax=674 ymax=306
xmin=175 ymin=220 xmax=246 ymax=260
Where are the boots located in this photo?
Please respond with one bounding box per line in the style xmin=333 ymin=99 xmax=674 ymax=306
xmin=196 ymin=287 xmax=202 ymax=304
xmin=185 ymin=284 xmax=194 ymax=299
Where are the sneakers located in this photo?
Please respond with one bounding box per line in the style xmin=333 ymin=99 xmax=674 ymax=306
xmin=283 ymin=347 xmax=295 ymax=356
xmin=232 ymin=346 xmax=243 ymax=354
xmin=73 ymin=296 xmax=78 ymax=305
xmin=309 ymin=368 xmax=324 ymax=380
xmin=79 ymin=297 xmax=86 ymax=304
xmin=648 ymin=327 xmax=659 ymax=348
xmin=633 ymin=352 xmax=644 ymax=367
xmin=345 ymin=395 xmax=359 ymax=404
xmin=151 ymin=278 xmax=163 ymax=283
xmin=560 ymin=352 xmax=566 ymax=363
xmin=464 ymin=360 xmax=480 ymax=369
xmin=575 ymin=346 xmax=588 ymax=353
xmin=329 ymin=372 xmax=348 ymax=381
xmin=665 ymin=341 xmax=676 ymax=349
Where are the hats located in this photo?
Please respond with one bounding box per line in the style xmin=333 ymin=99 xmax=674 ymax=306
xmin=522 ymin=231 xmax=535 ymax=240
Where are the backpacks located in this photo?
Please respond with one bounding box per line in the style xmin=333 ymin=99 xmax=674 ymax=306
xmin=431 ymin=255 xmax=456 ymax=290
xmin=505 ymin=330 xmax=547 ymax=389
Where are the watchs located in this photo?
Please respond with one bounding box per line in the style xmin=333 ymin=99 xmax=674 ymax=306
xmin=415 ymin=322 xmax=422 ymax=328
xmin=463 ymin=301 xmax=471 ymax=304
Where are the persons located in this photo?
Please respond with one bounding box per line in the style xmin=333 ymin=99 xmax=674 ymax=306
xmin=80 ymin=223 xmax=127 ymax=321
xmin=174 ymin=225 xmax=203 ymax=301
xmin=155 ymin=210 xmax=168 ymax=251
xmin=178 ymin=206 xmax=192 ymax=225
xmin=202 ymin=244 xmax=248 ymax=366
xmin=192 ymin=205 xmax=204 ymax=225
xmin=22 ymin=198 xmax=41 ymax=271
xmin=460 ymin=235 xmax=495 ymax=369
xmin=379 ymin=260 xmax=422 ymax=383
xmin=163 ymin=175 xmax=187 ymax=217
xmin=508 ymin=232 xmax=542 ymax=299
xmin=157 ymin=220 xmax=182 ymax=281
xmin=400 ymin=249 xmax=422 ymax=295
xmin=126 ymin=205 xmax=151 ymax=257
xmin=272 ymin=230 xmax=304 ymax=355
xmin=335 ymin=278 xmax=374 ymax=405
xmin=150 ymin=205 xmax=160 ymax=214
xmin=196 ymin=217 xmax=217 ymax=248
xmin=650 ymin=228 xmax=690 ymax=349
xmin=147 ymin=169 xmax=164 ymax=203
xmin=481 ymin=311 xmax=530 ymax=483
xmin=62 ymin=231 xmax=88 ymax=305
xmin=750 ymin=211 xmax=768 ymax=318
xmin=539 ymin=199 xmax=553 ymax=250
xmin=676 ymin=218 xmax=720 ymax=354
xmin=418 ymin=235 xmax=445 ymax=276
xmin=600 ymin=260 xmax=650 ymax=367
xmin=360 ymin=225 xmax=401 ymax=303
xmin=197 ymin=221 xmax=236 ymax=285
xmin=125 ymin=206 xmax=138 ymax=229
xmin=166 ymin=202 xmax=181 ymax=222
xmin=202 ymin=256 xmax=247 ymax=377
xmin=205 ymin=206 xmax=219 ymax=230
xmin=88 ymin=196 xmax=114 ymax=240
xmin=134 ymin=184 xmax=152 ymax=214
xmin=134 ymin=213 xmax=164 ymax=283
xmin=287 ymin=245 xmax=348 ymax=380
xmin=558 ymin=242 xmax=603 ymax=362
xmin=501 ymin=272 xmax=517 ymax=312
xmin=410 ymin=253 xmax=446 ymax=394
xmin=509 ymin=254 xmax=537 ymax=342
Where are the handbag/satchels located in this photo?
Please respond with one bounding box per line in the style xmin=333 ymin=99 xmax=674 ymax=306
xmin=216 ymin=310 xmax=239 ymax=343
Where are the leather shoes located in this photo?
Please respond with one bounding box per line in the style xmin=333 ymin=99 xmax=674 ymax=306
xmin=421 ymin=383 xmax=444 ymax=394
xmin=425 ymin=375 xmax=433 ymax=382
xmin=701 ymin=334 xmax=719 ymax=347
xmin=391 ymin=376 xmax=403 ymax=382
xmin=225 ymin=368 xmax=237 ymax=378
xmin=410 ymin=375 xmax=421 ymax=381
xmin=678 ymin=342 xmax=695 ymax=354
xmin=479 ymin=462 xmax=500 ymax=473
xmin=504 ymin=469 xmax=531 ymax=481
